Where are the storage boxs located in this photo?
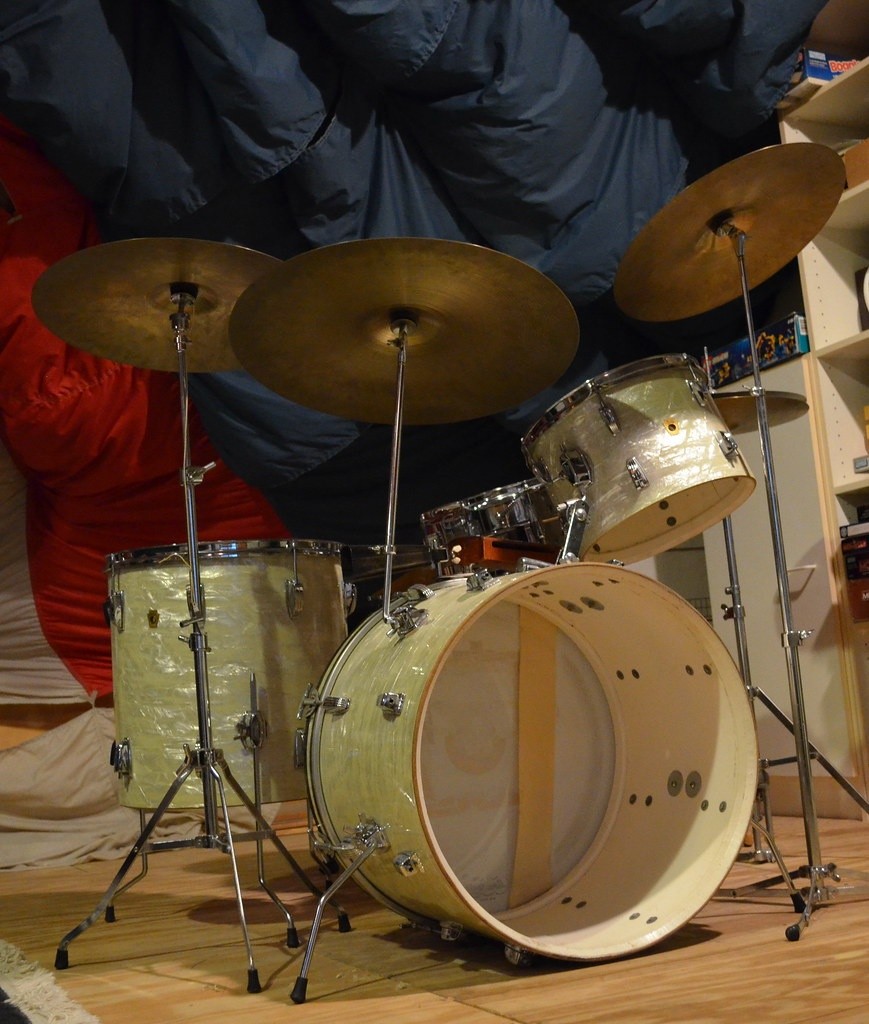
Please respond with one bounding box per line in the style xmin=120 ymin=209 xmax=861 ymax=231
xmin=843 ymin=138 xmax=869 ymax=191
xmin=700 ymin=309 xmax=808 ymax=391
xmin=784 ymin=45 xmax=866 ymax=100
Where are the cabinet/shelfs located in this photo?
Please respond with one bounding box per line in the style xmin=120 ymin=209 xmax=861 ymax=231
xmin=771 ymin=0 xmax=869 ymax=827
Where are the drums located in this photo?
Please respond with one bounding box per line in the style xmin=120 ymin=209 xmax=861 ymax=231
xmin=311 ymin=559 xmax=766 ymax=961
xmin=103 ymin=534 xmax=355 ymax=812
xmin=519 ymin=350 xmax=758 ymax=567
xmin=419 ymin=478 xmax=565 ymax=580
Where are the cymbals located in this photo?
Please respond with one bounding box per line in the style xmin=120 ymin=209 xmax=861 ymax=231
xmin=30 ymin=234 xmax=289 ymax=377
xmin=227 ymin=232 xmax=584 ymax=427
xmin=712 ymin=386 xmax=810 ymax=436
xmin=610 ymin=139 xmax=850 ymax=326
